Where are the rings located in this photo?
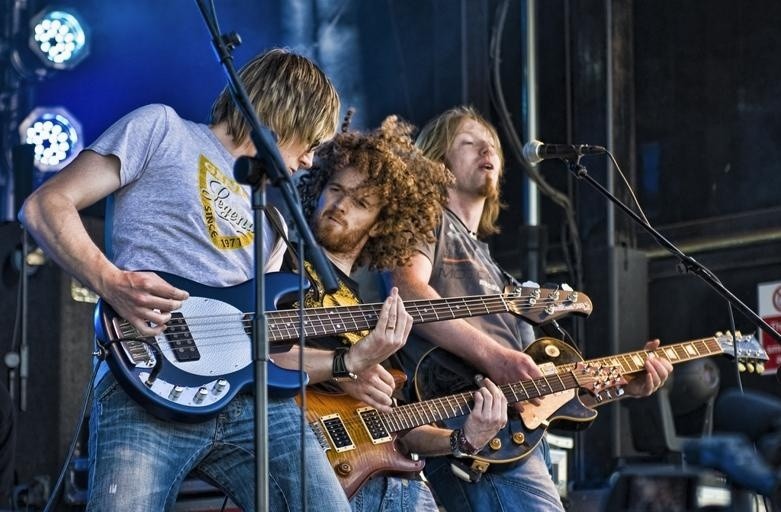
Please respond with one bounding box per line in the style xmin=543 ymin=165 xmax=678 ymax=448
xmin=385 ymin=325 xmax=396 ymax=331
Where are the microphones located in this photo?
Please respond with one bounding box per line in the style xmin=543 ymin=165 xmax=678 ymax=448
xmin=521 ymin=139 xmax=605 ymax=167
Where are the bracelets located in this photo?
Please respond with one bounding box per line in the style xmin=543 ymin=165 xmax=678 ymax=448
xmin=331 ymin=345 xmax=359 ymax=383
xmin=451 ymin=426 xmax=480 ymax=459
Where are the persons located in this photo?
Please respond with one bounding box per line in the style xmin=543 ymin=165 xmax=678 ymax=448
xmin=275 ymin=127 xmax=512 ymax=511
xmin=383 ymin=105 xmax=677 ymax=511
xmin=12 ymin=44 xmax=415 ymax=510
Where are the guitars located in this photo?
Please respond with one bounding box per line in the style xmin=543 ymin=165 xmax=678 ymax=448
xmin=414 ymin=329 xmax=771 ymax=475
xmin=94 ymin=270 xmax=593 ymax=425
xmin=294 ymin=363 xmax=628 ymax=504
